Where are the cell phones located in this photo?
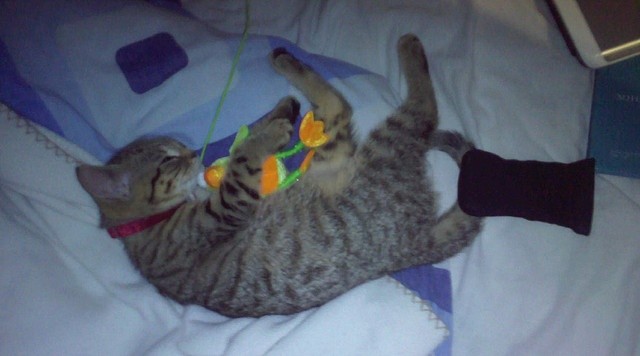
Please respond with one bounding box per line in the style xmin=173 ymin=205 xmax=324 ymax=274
xmin=547 ymin=0 xmax=640 ymax=71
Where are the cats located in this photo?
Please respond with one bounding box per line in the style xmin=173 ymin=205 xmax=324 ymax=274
xmin=76 ymin=33 xmax=486 ymax=317
